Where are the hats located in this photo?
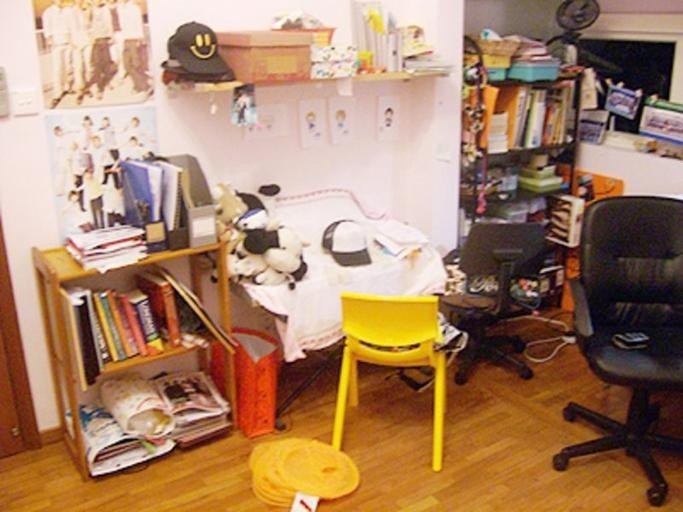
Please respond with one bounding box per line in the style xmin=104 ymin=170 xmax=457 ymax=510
xmin=167 ymin=22 xmax=229 ymax=75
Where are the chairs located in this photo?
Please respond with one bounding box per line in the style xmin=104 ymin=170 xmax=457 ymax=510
xmin=444 ymin=221 xmax=546 ymax=386
xmin=556 ymin=192 xmax=683 ymax=506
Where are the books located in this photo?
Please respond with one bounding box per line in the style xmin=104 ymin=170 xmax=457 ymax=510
xmin=466 ymin=27 xmax=579 ymax=157
xmin=67 ymin=161 xmax=183 ymax=273
xmin=351 ymin=0 xmax=455 ymax=77
xmin=61 ymin=266 xmax=239 ymax=386
xmin=65 ymin=373 xmax=231 ymax=461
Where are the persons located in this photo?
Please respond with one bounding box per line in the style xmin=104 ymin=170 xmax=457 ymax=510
xmin=40 ymin=1 xmax=152 ymax=111
xmin=52 ymin=114 xmax=145 ymax=229
xmin=235 ymin=88 xmax=249 ymax=127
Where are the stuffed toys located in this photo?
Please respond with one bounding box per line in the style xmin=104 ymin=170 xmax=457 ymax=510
xmin=207 ymin=180 xmax=313 ymax=292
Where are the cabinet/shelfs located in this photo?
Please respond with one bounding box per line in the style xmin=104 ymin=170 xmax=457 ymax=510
xmin=26 ymin=220 xmax=238 ymax=486
xmin=463 ymin=28 xmax=582 ymax=321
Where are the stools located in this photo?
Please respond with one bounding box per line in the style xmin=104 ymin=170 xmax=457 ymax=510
xmin=329 ymin=290 xmax=444 ymax=472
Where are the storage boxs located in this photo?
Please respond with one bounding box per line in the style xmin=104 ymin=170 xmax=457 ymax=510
xmin=208 ymin=28 xmax=309 ymax=86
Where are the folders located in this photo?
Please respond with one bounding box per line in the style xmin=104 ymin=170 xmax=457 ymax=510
xmin=119 ymin=156 xmax=165 ymax=222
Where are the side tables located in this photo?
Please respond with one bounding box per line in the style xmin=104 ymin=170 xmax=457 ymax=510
xmin=217 ymin=193 xmax=443 ymax=435
